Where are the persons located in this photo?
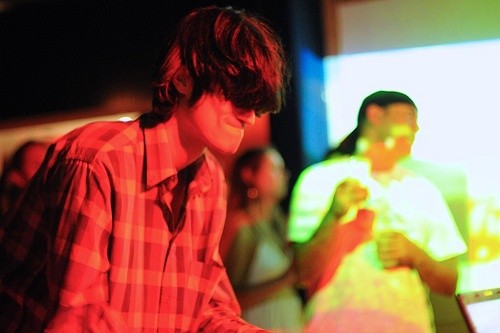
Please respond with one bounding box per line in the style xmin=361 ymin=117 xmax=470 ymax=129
xmin=287 ymin=89 xmax=468 ymax=333
xmin=0 ymin=5 xmax=283 ymax=333
xmin=218 ymin=140 xmax=305 ymax=333
